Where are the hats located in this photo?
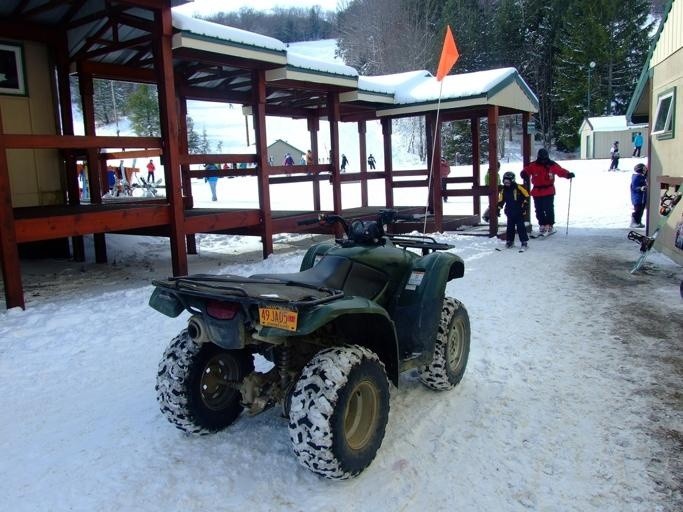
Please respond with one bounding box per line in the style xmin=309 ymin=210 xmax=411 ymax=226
xmin=634 ymin=164 xmax=646 ymax=174
xmin=502 ymin=171 xmax=516 ymax=186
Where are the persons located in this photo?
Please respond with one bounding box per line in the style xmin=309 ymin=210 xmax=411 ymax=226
xmin=204 ymin=150 xmax=312 ymax=201
xmin=632 ymin=132 xmax=643 ymax=157
xmin=482 ymin=162 xmax=502 ymax=222
xmin=368 ymin=153 xmax=376 ymax=170
xmin=608 ymin=149 xmax=619 ymax=171
xmin=498 ymin=171 xmax=529 ymax=247
xmin=630 ymin=163 xmax=648 ymax=228
xmin=117 ymin=160 xmax=130 ymax=196
xmin=441 ymin=157 xmax=451 ymax=202
xmin=340 ymin=154 xmax=349 ymax=172
xmin=520 ymin=148 xmax=575 ymax=232
xmin=610 ymin=141 xmax=619 ymax=159
xmin=146 ymin=160 xmax=155 ymax=183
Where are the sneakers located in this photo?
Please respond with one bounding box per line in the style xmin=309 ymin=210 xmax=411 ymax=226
xmin=539 ymin=224 xmax=553 ymax=232
xmin=522 ymin=241 xmax=528 ymax=247
xmin=506 ymin=240 xmax=514 ymax=247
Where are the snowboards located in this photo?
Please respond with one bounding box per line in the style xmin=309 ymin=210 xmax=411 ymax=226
xmin=628 ymin=183 xmax=683 ymax=273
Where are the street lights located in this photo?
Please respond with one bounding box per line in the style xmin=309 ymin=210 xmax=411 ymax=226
xmin=587 ymin=61 xmax=595 ymax=118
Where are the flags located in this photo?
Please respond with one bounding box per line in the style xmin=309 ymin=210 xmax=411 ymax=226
xmin=436 ymin=26 xmax=460 ymax=81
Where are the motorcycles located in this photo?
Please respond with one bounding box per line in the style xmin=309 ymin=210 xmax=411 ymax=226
xmin=150 ymin=202 xmax=475 ymax=482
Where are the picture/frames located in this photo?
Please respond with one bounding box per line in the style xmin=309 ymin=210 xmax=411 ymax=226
xmin=0 ymin=38 xmax=31 ymax=99
